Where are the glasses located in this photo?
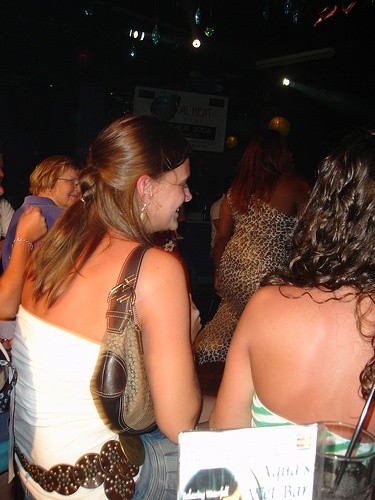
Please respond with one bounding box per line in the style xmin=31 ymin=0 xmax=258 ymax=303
xmin=57 ymin=178 xmax=80 ymax=186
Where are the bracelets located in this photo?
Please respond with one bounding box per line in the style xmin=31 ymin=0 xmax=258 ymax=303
xmin=10 ymin=238 xmax=34 ymax=250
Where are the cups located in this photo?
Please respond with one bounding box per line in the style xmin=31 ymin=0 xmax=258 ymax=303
xmin=313 ymin=422 xmax=375 ymax=500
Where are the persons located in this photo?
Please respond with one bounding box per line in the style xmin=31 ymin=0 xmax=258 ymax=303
xmin=0 ymin=152 xmax=15 ymax=259
xmin=207 ymin=135 xmax=375 ymax=500
xmin=10 ymin=115 xmax=215 ymax=500
xmin=193 ymin=111 xmax=319 ymax=370
xmin=1 ymin=155 xmax=81 ymax=272
xmin=210 ymin=192 xmax=227 ymax=260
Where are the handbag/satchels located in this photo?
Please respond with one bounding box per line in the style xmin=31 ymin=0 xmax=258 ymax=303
xmin=89 ymin=241 xmax=199 ymax=437
xmin=0 ymin=340 xmax=18 ymax=475
